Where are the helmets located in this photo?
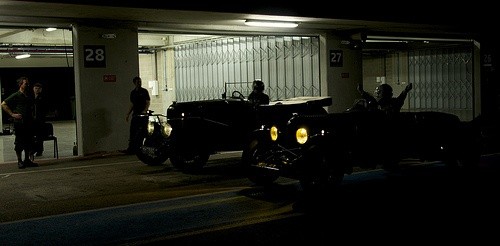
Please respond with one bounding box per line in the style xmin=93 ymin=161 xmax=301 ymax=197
xmin=374 ymin=84 xmax=393 ymax=104
xmin=253 ymin=81 xmax=264 ymax=93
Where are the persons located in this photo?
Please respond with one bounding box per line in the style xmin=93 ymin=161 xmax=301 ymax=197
xmin=124 ymin=77 xmax=150 ymax=153
xmin=1 ymin=77 xmax=39 ymax=170
xmin=31 ymin=83 xmax=48 ymax=156
xmin=375 ymin=83 xmax=413 ymax=113
xmin=248 ymin=80 xmax=269 ymax=105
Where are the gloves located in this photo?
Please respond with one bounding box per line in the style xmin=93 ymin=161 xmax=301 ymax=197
xmin=405 ymin=83 xmax=412 ymax=92
xmin=359 ymin=83 xmax=363 ymax=94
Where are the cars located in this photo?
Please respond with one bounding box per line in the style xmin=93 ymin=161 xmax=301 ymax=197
xmin=240 ymin=83 xmax=467 ymax=195
xmin=134 ymin=82 xmax=333 ymax=173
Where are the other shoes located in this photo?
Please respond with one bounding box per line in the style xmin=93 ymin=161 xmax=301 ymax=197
xmin=17 ymin=160 xmax=25 ymax=168
xmin=24 ymin=159 xmax=38 ymax=167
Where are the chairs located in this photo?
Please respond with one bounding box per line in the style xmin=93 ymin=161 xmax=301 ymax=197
xmin=34 ymin=123 xmax=59 ymax=160
